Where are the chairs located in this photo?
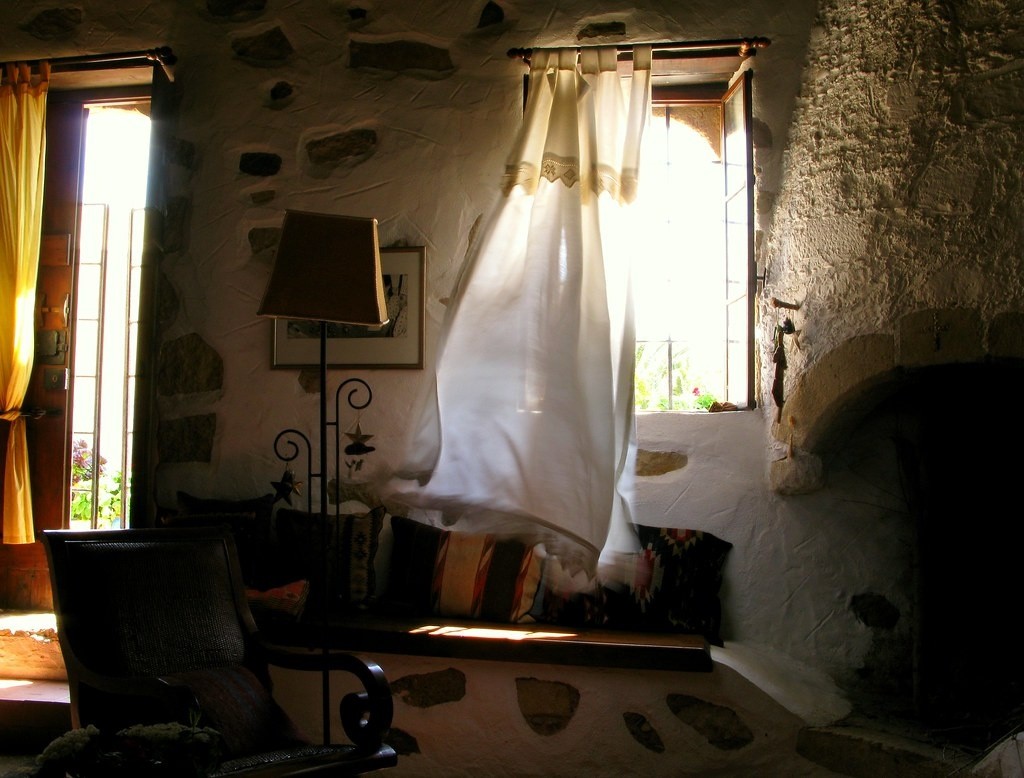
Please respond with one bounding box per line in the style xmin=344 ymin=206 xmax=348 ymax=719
xmin=37 ymin=524 xmax=397 ymax=778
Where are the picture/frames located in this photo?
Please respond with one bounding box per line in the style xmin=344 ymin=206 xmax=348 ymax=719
xmin=271 ymin=244 xmax=428 ymax=372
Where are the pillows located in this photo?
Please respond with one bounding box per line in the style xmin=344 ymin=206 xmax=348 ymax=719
xmin=380 ymin=516 xmax=557 ymax=629
xmin=158 ymin=663 xmax=314 ymax=763
xmin=254 ymin=503 xmax=387 ymax=614
xmin=594 ymin=522 xmax=734 ymax=649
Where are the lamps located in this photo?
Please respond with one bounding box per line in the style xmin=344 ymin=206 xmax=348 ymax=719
xmin=254 ymin=207 xmax=393 ymax=621
